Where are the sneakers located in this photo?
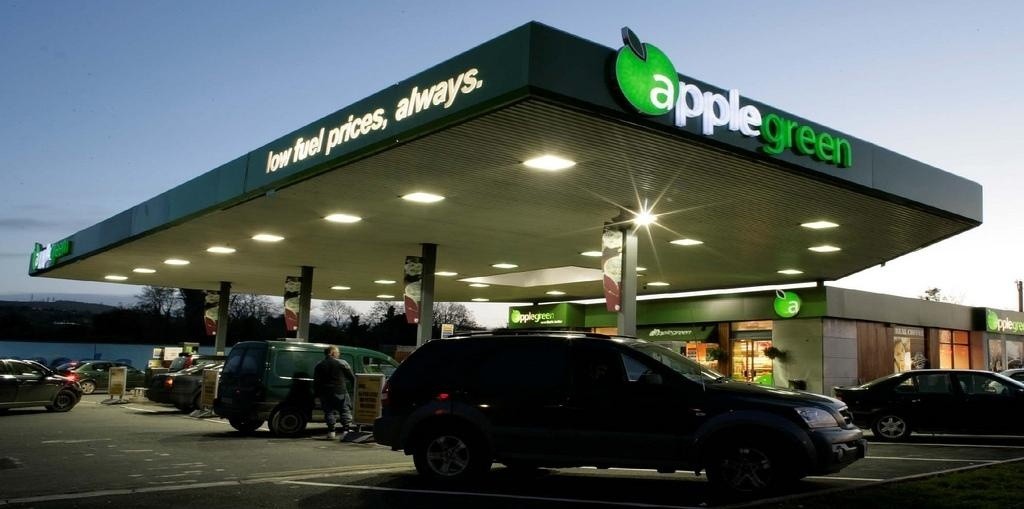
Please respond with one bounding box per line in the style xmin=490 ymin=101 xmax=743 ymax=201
xmin=328 ymin=432 xmax=336 ymax=438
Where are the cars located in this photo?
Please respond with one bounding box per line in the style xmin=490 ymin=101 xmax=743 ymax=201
xmin=374 ymin=330 xmax=868 ymax=491
xmin=832 ymin=368 xmax=1024 ymax=442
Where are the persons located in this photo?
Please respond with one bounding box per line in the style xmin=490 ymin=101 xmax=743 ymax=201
xmin=313 ymin=345 xmax=355 ymax=440
xmin=182 ymin=353 xmax=193 ymax=369
xmin=167 ymin=353 xmax=186 ymax=372
